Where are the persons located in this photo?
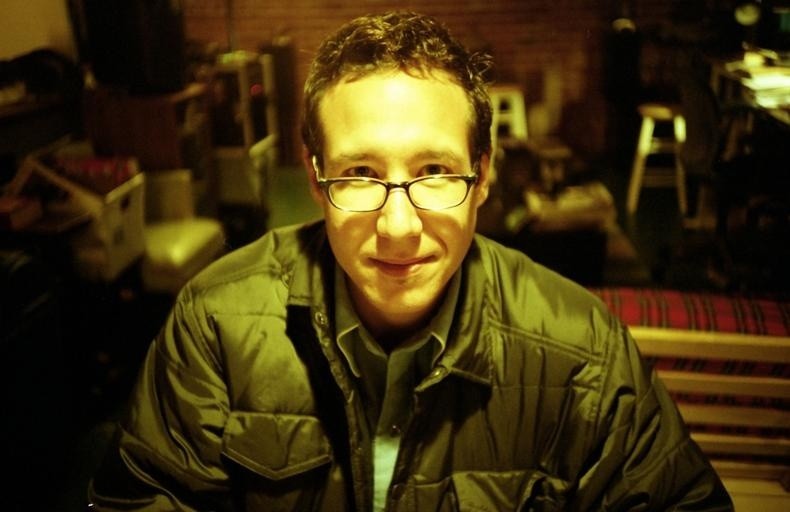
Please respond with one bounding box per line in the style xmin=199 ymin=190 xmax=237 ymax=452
xmin=88 ymin=8 xmax=737 ymax=511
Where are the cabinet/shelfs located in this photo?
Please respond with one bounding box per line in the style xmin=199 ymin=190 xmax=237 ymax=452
xmin=86 ymin=83 xmax=218 ymax=220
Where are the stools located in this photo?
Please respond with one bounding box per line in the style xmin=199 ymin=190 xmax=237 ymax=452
xmin=625 ymin=102 xmax=687 ymax=214
xmin=488 ymin=84 xmax=528 ymax=181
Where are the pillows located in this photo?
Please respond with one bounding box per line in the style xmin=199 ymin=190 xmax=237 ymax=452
xmin=39 ymin=150 xmax=138 ymax=206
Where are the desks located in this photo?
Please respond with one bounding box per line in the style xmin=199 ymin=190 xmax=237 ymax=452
xmin=696 ymin=57 xmax=790 ymax=217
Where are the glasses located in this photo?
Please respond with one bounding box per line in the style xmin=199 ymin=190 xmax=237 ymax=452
xmin=310 ymin=151 xmax=481 ymax=211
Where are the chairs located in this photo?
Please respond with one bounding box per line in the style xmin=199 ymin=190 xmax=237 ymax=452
xmin=48 ymin=169 xmax=225 ymax=294
xmin=589 ymin=285 xmax=790 ymax=512
